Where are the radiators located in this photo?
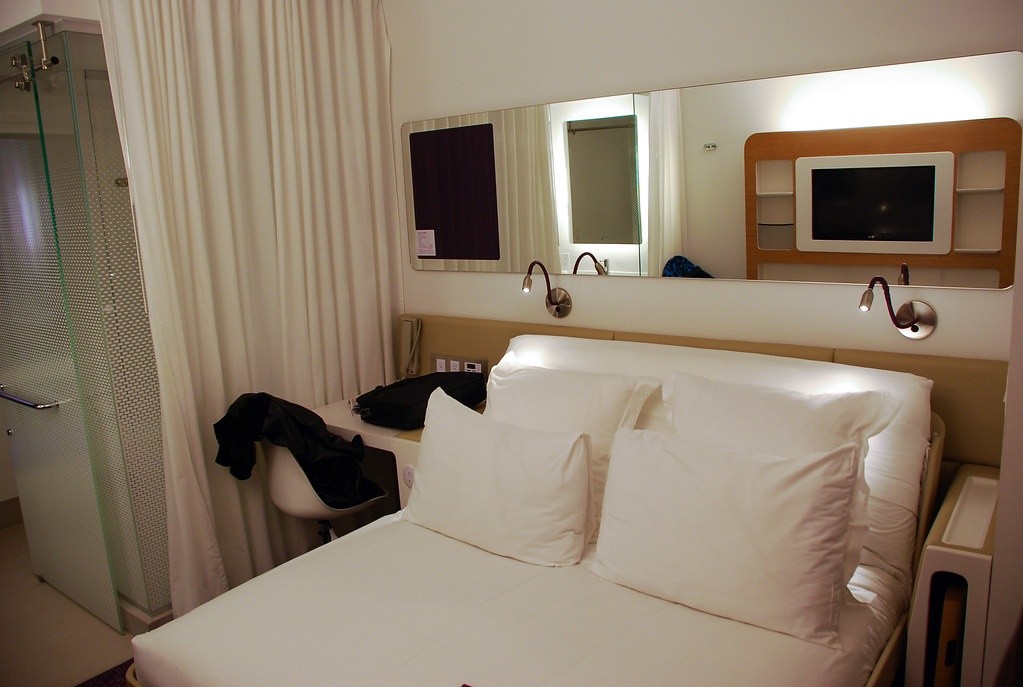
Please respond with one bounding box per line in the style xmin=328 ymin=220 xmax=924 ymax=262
xmin=899 ymin=463 xmax=1000 ymax=687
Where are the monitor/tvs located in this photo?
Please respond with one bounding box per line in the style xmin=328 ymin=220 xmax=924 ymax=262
xmin=796 ymin=152 xmax=954 ymax=253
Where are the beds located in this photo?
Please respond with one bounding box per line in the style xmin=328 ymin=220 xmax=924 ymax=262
xmin=130 ymin=335 xmax=947 ymax=687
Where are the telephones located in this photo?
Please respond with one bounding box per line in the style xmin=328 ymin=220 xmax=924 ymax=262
xmin=399 ymin=317 xmax=422 ymax=376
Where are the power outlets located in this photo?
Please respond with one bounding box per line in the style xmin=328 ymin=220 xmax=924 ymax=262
xmin=429 ymin=352 xmax=488 ymax=374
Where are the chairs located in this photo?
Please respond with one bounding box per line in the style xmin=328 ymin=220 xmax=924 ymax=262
xmin=258 ymin=436 xmax=392 ymax=543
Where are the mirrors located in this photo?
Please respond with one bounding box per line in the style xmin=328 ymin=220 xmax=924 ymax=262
xmin=401 ymin=49 xmax=1023 ymax=291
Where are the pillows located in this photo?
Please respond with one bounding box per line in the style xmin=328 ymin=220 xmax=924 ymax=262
xmin=403 ymin=387 xmax=596 ymax=566
xmin=658 ymin=371 xmax=904 ymax=605
xmin=487 ymin=362 xmax=662 ymax=540
xmin=587 ymin=427 xmax=863 ymax=652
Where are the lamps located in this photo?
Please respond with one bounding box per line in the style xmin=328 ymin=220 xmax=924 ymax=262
xmin=573 ymin=252 xmax=608 ymax=275
xmin=522 ymin=260 xmax=572 ymax=320
xmin=858 ymin=276 xmax=937 ymax=340
xmin=897 ymin=263 xmax=910 ymax=286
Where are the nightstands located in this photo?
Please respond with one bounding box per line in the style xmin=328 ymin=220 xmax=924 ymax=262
xmin=314 ymin=385 xmax=487 ymax=510
